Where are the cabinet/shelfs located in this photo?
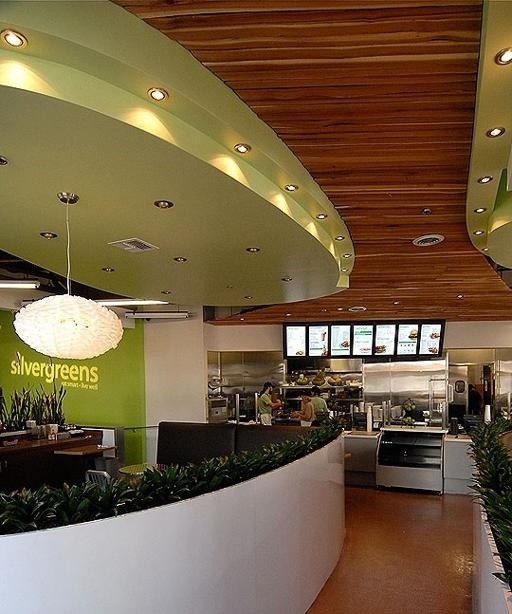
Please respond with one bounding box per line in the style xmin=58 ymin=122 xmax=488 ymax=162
xmin=280 ymin=382 xmax=364 ymax=415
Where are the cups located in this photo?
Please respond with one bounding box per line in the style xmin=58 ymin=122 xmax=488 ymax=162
xmin=47 ymin=423 xmax=57 ymax=440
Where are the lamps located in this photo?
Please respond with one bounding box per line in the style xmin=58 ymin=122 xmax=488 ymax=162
xmin=13 ymin=192 xmax=124 ymax=361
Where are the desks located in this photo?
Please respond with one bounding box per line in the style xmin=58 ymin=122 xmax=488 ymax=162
xmin=117 ymin=463 xmax=170 ymax=481
xmin=52 ymin=444 xmax=121 ymax=481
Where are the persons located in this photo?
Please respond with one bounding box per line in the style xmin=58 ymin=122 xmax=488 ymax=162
xmin=257 ymin=381 xmax=284 ymax=426
xmin=291 ymin=391 xmax=315 ymax=427
xmin=310 ymin=386 xmax=329 ymax=415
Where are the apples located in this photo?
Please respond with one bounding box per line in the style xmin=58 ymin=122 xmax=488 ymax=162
xmin=404 ymin=402 xmax=416 ymax=411
xmin=403 ymin=416 xmax=415 ymax=424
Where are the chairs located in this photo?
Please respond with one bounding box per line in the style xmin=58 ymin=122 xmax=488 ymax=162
xmin=85 ymin=470 xmax=119 ymax=493
